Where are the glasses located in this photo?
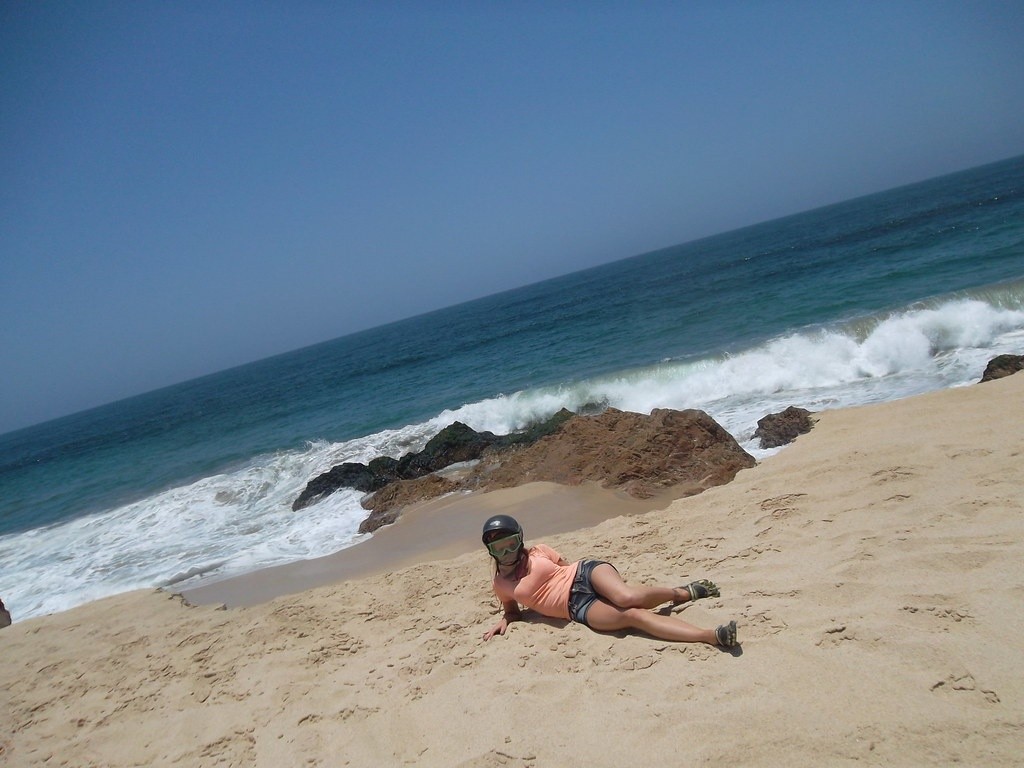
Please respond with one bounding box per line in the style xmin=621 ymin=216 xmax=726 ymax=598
xmin=488 ymin=531 xmax=522 ymax=557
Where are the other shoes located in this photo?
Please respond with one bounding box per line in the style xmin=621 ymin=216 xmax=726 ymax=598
xmin=715 ymin=621 xmax=736 ymax=648
xmin=670 ymin=579 xmax=720 ymax=605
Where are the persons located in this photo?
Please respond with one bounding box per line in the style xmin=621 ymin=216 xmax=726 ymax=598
xmin=481 ymin=515 xmax=737 ymax=646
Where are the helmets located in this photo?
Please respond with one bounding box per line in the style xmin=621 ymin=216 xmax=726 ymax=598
xmin=482 ymin=515 xmax=523 ymax=544
